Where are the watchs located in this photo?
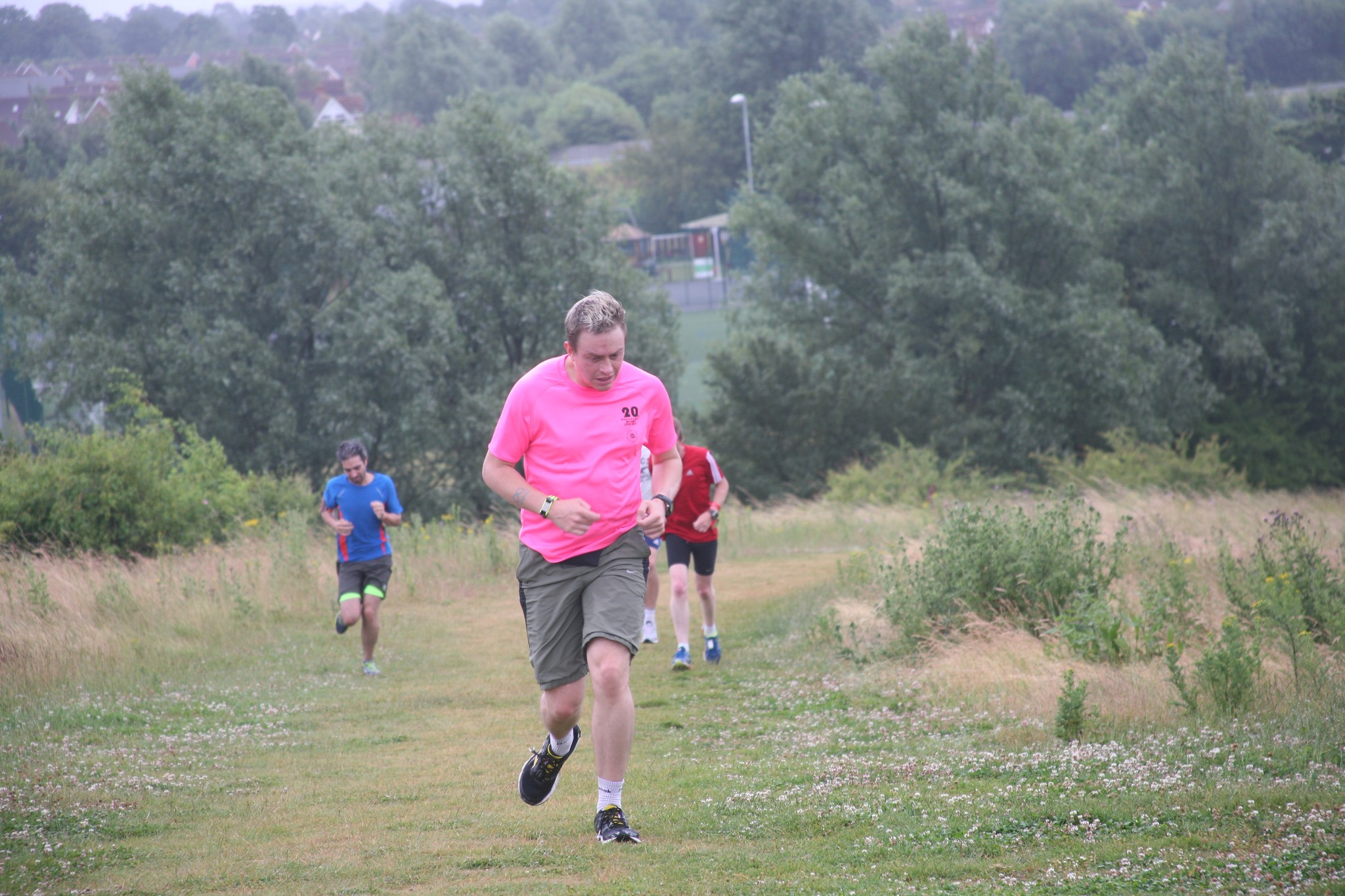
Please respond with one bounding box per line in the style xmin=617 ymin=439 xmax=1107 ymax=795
xmin=652 ymin=494 xmax=675 ymax=516
xmin=709 ymin=508 xmax=719 ymax=520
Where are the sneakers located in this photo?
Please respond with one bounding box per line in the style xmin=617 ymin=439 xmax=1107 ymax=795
xmin=594 ymin=804 xmax=640 ymax=844
xmin=641 ymin=626 xmax=659 ymax=645
xmin=362 ymin=659 xmax=379 ymax=676
xmin=518 ymin=723 xmax=581 ymax=807
xmin=672 ymin=649 xmax=692 ymax=672
xmin=335 ymin=613 xmax=349 ymax=634
xmin=702 ymin=627 xmax=722 ymax=664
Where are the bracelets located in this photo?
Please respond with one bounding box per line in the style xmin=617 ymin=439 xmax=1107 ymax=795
xmin=539 ymin=495 xmax=558 ymax=517
xmin=707 ymin=501 xmax=721 ymax=510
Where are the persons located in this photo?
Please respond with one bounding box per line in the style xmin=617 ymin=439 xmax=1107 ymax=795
xmin=633 ymin=417 xmax=728 ymax=671
xmin=319 ymin=439 xmax=404 ymax=677
xmin=481 ymin=290 xmax=683 ymax=846
